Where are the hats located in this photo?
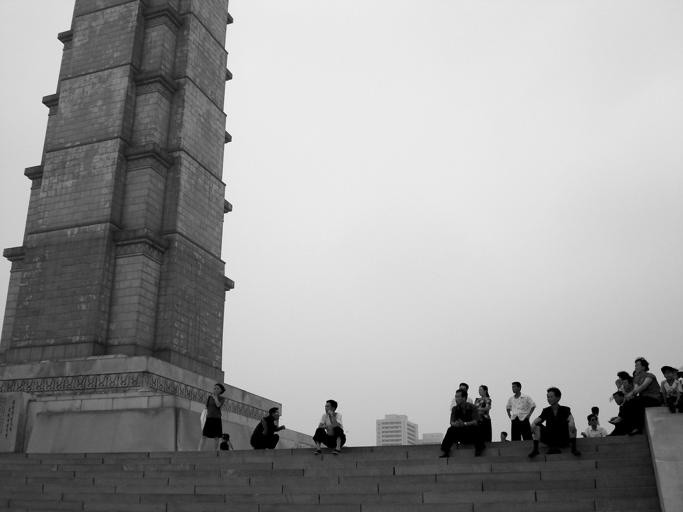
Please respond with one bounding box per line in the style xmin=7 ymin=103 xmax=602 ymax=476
xmin=662 ymin=366 xmax=679 ymax=373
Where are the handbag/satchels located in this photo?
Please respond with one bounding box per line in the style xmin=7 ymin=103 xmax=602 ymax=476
xmin=200 ymin=408 xmax=207 ymax=431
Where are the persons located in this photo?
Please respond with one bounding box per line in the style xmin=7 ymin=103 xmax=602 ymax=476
xmin=312 ymin=399 xmax=346 ymax=455
xmin=438 ymin=356 xmax=683 ymax=458
xmin=249 ymin=407 xmax=287 ymax=449
xmin=196 ymin=383 xmax=226 ymax=451
